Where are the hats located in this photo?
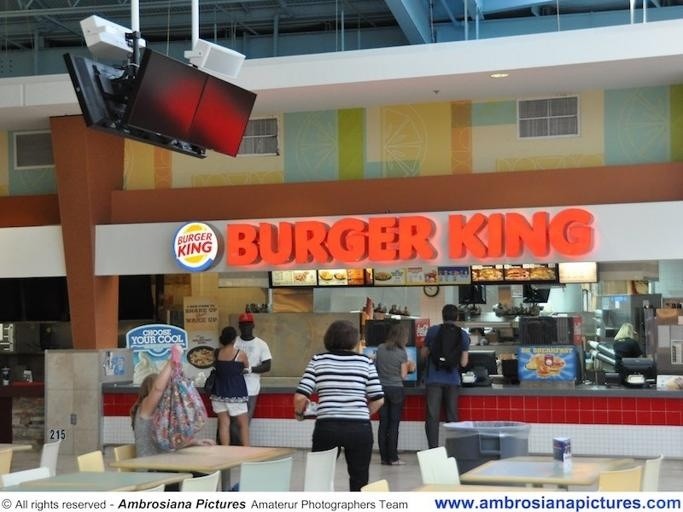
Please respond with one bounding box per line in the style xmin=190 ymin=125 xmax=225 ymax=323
xmin=239 ymin=313 xmax=254 ymax=324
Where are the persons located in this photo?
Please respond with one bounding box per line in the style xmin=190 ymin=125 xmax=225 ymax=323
xmin=215 ymin=312 xmax=272 ymax=446
xmin=293 ymin=321 xmax=385 ymax=492
xmin=375 ymin=323 xmax=410 ymax=465
xmin=421 ymin=304 xmax=470 ymax=450
xmin=130 ymin=342 xmax=184 ymax=472
xmin=207 ymin=326 xmax=249 ymax=446
xmin=612 ymin=323 xmax=643 ymax=386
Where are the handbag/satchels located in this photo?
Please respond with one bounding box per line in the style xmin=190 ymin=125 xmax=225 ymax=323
xmin=151 ymin=371 xmax=209 ymax=453
xmin=431 ymin=327 xmax=459 ymax=371
xmin=205 ymin=369 xmax=216 ymax=394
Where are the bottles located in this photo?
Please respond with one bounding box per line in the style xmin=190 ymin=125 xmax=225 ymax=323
xmin=375 ymin=302 xmax=409 ymax=315
xmin=245 ymin=303 xmax=267 ymax=312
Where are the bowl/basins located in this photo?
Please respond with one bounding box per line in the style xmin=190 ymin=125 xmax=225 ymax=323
xmin=185 ymin=346 xmax=216 ymax=368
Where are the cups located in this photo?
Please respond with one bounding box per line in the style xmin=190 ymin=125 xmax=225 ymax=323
xmin=0 ymin=365 xmax=10 ymax=386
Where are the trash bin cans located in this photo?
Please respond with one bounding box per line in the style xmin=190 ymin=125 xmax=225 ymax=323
xmin=443 ymin=421 xmax=531 ymax=487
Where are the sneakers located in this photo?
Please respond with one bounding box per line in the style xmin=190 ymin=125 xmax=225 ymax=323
xmin=381 ymin=460 xmax=407 ymax=465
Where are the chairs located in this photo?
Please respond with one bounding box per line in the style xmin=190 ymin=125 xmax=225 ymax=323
xmin=0 ymin=430 xmax=668 ymax=490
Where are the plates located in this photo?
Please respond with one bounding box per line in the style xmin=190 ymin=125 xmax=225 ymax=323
xmin=375 ymin=273 xmax=391 ymax=281
xmin=320 ymin=272 xmax=345 ymax=280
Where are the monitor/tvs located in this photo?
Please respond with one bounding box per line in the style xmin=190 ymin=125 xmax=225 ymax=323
xmin=620 ymin=357 xmax=656 ymax=386
xmin=524 ymin=285 xmax=551 ymax=303
xmin=123 ymin=49 xmax=257 ymax=159
xmin=457 ymin=285 xmax=486 ymax=305
xmin=62 ymin=50 xmax=206 ymax=158
xmin=467 ymin=351 xmax=498 ymax=376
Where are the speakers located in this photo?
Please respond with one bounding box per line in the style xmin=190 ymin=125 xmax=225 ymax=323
xmin=80 ymin=13 xmax=144 ymax=59
xmin=189 ymin=38 xmax=246 ymax=79
